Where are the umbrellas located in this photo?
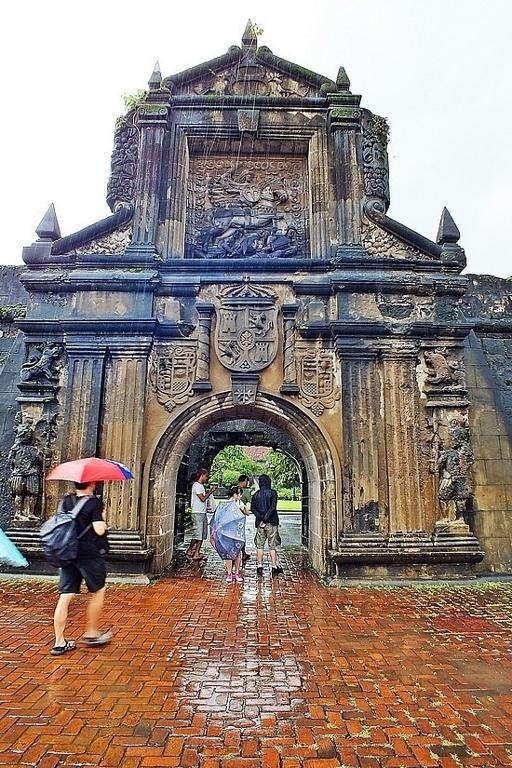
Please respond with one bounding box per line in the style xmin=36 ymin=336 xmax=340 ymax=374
xmin=45 ymin=456 xmax=136 ymax=484
xmin=207 ymin=500 xmax=246 ymax=561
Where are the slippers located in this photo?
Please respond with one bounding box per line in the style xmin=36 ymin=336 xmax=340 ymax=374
xmin=243 ymin=555 xmax=249 ymax=560
xmin=185 ymin=552 xmax=207 ymax=562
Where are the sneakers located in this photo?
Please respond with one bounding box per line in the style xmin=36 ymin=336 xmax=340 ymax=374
xmin=257 ymin=566 xmax=283 ymax=575
xmin=84 ymin=632 xmax=111 ymax=646
xmin=226 ymin=573 xmax=244 ymax=582
xmin=51 ymin=640 xmax=76 ymax=655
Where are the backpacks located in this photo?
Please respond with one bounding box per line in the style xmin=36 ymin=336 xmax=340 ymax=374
xmin=40 ymin=496 xmax=100 ymax=567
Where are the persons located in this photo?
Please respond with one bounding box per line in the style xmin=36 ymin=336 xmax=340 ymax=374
xmin=185 ymin=468 xmax=218 ymax=560
xmin=229 ymin=475 xmax=252 ymax=563
xmin=50 ymin=480 xmax=114 ymax=655
xmin=7 ymin=428 xmax=46 ymax=522
xmin=225 ymin=486 xmax=250 ymax=583
xmin=251 ymin=473 xmax=283 ymax=573
xmin=433 ymin=427 xmax=472 ymax=526
